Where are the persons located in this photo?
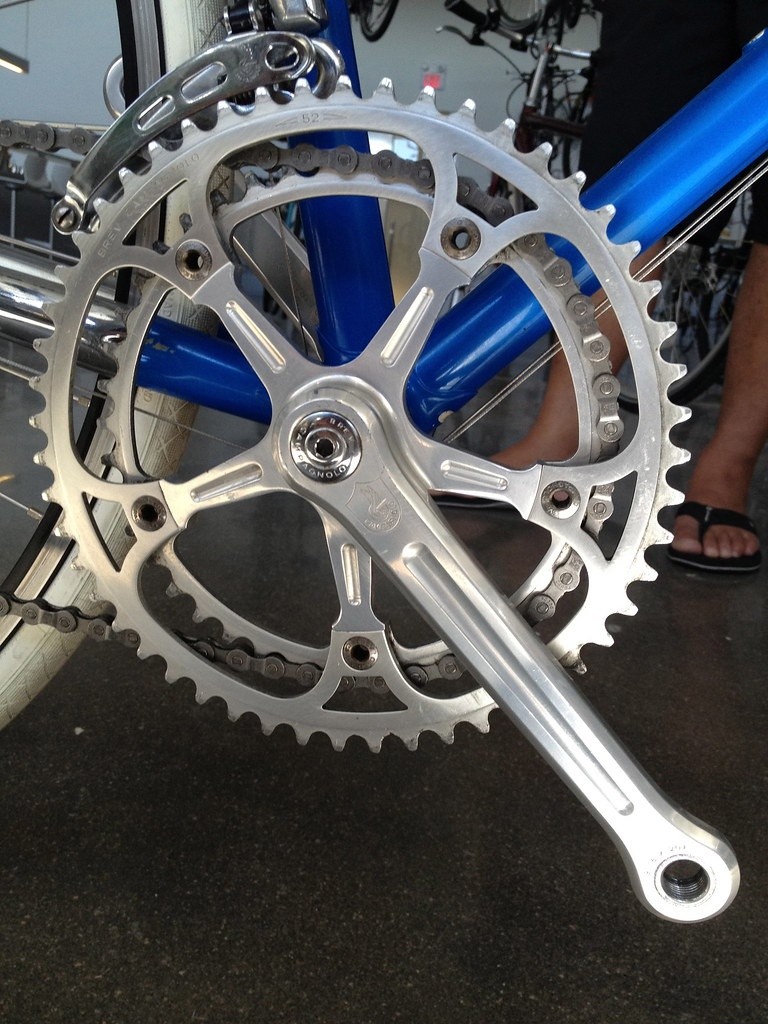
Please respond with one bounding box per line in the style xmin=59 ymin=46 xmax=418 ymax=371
xmin=428 ymin=0 xmax=768 ymax=572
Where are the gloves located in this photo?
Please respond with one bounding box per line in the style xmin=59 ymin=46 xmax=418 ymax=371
xmin=541 ymin=0 xmax=583 ymax=36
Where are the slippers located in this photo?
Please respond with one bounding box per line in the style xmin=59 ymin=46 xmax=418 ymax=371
xmin=430 ymin=492 xmax=595 ymax=525
xmin=667 ymin=500 xmax=763 ymax=577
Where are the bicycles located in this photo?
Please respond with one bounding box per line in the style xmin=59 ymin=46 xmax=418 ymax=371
xmin=346 ymin=0 xmax=401 ymax=45
xmin=1 ymin=0 xmax=768 ymax=927
xmin=436 ymin=0 xmax=757 ymax=415
